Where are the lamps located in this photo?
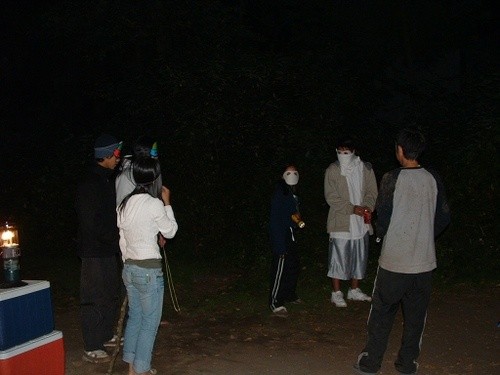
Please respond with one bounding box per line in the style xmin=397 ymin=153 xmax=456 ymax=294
xmin=0 ymin=220 xmax=29 ymax=289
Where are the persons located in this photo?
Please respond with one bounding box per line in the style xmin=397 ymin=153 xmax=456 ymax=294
xmin=324 ymin=138 xmax=379 ymax=307
xmin=78 ymin=134 xmax=178 ymax=375
xmin=269 ymin=163 xmax=305 ymax=318
xmin=351 ymin=129 xmax=438 ymax=375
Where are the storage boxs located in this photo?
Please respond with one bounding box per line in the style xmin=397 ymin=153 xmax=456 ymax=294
xmin=0 ymin=280 xmax=55 ymax=352
xmin=0 ymin=330 xmax=66 ymax=375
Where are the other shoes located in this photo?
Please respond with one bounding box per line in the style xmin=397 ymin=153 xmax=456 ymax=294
xmin=400 ymin=372 xmax=415 ymax=375
xmin=290 ymin=297 xmax=304 ymax=304
xmin=136 ymin=369 xmax=157 ymax=375
xmin=352 ymin=365 xmax=376 ymax=375
xmin=273 ymin=306 xmax=288 ymax=317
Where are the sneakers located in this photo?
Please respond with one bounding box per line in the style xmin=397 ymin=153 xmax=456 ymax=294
xmin=103 ymin=334 xmax=124 ymax=347
xmin=330 ymin=291 xmax=348 ymax=307
xmin=346 ymin=287 xmax=372 ymax=301
xmin=82 ymin=349 xmax=111 ymax=363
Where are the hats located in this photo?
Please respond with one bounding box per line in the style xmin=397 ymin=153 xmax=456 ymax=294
xmin=94 ymin=135 xmax=122 ymax=157
